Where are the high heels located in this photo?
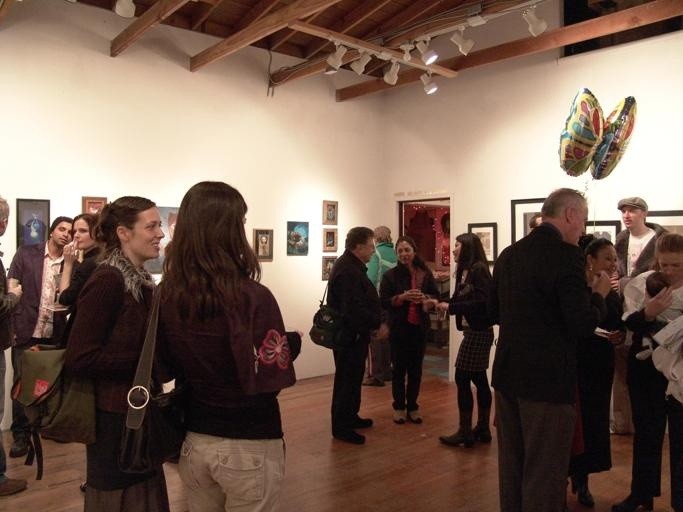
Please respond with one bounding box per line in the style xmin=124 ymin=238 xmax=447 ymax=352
xmin=572 ymin=480 xmax=594 ymax=506
xmin=611 ymin=493 xmax=653 ymax=512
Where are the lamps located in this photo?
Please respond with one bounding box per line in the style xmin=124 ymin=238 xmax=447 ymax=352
xmin=400 ymin=44 xmax=414 ymax=62
xmin=115 ymin=0 xmax=135 ymax=18
xmin=288 ymin=19 xmax=458 ymax=95
xmin=413 ymin=0 xmax=548 ymax=66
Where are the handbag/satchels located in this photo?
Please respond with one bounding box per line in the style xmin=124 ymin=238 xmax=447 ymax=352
xmin=309 ymin=304 xmax=360 ymax=350
xmin=119 ymin=383 xmax=185 ymax=473
xmin=10 ymin=344 xmax=96 ymax=444
xmin=458 ymin=277 xmax=501 ymax=331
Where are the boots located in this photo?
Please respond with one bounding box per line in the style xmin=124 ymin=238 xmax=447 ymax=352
xmin=472 ymin=389 xmax=492 ymax=442
xmin=439 ymin=391 xmax=474 ymax=447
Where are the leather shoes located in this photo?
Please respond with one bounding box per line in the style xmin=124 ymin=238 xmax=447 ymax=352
xmin=9 ymin=438 xmax=28 ymax=457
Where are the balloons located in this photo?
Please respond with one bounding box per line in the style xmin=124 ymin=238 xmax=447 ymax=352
xmin=557 ymin=85 xmax=637 ymax=180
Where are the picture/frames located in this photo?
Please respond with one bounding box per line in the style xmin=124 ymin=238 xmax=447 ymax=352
xmin=253 ymin=228 xmax=273 ymax=262
xmin=644 ymin=209 xmax=683 ymax=234
xmin=321 ymin=256 xmax=337 ymax=281
xmin=585 ymin=220 xmax=621 ymax=250
xmin=322 ymin=200 xmax=338 ymax=225
xmin=15 ymin=198 xmax=50 ymax=249
xmin=467 ymin=222 xmax=498 ymax=266
xmin=510 ymin=198 xmax=547 ymax=245
xmin=322 ymin=228 xmax=337 ymax=252
xmin=82 ymin=197 xmax=108 ymax=217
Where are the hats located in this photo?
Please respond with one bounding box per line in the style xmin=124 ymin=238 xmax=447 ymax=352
xmin=618 ymin=196 xmax=648 ymax=212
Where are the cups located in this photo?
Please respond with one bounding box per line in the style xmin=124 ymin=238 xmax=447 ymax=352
xmin=411 ymin=289 xmax=421 ymax=304
xmin=5 ymin=278 xmax=18 ymax=294
xmin=436 ymin=309 xmax=447 ymax=321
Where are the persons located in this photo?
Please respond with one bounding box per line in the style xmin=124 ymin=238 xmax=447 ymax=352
xmin=5 ymin=217 xmax=75 ymax=457
xmin=327 ymin=227 xmax=388 ymax=444
xmin=569 ymin=197 xmax=682 ymax=512
xmin=146 ymin=181 xmax=303 ymax=512
xmin=362 ymin=226 xmax=440 ymax=423
xmin=63 ymin=196 xmax=182 ymax=512
xmin=58 ymin=213 xmax=102 ymax=349
xmin=0 ymin=197 xmax=28 ymax=494
xmin=259 ymin=235 xmax=269 ymax=256
xmin=490 ymin=187 xmax=610 ymax=511
xmin=157 ymin=212 xmax=176 ymax=270
xmin=440 ymin=233 xmax=495 ymax=448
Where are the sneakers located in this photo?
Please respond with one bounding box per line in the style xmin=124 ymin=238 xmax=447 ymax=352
xmin=334 ymin=429 xmax=364 ymax=443
xmin=408 ymin=408 xmax=423 ymax=423
xmin=392 ymin=407 xmax=406 ymax=423
xmin=362 ymin=376 xmax=385 ymax=386
xmin=383 ymin=369 xmax=393 ymax=380
xmin=350 ymin=414 xmax=373 ymax=428
xmin=0 ymin=475 xmax=28 ymax=496
